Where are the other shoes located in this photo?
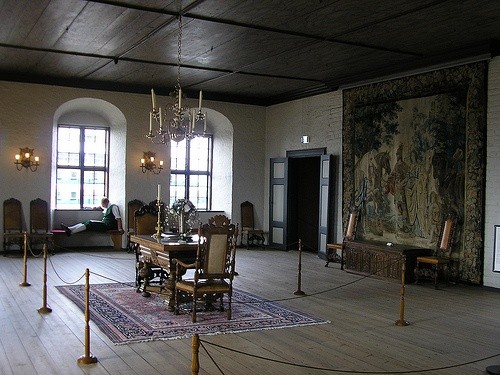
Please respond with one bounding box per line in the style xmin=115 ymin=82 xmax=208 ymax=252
xmin=60 ymin=223 xmax=72 ymax=237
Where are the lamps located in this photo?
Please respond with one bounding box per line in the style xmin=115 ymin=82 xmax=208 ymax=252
xmin=140 ymin=150 xmax=164 ymax=175
xmin=147 ymin=11 xmax=207 ymax=147
xmin=14 ymin=147 xmax=41 ymax=172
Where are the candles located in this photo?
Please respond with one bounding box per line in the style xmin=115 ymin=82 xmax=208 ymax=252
xmin=158 ymin=184 xmax=162 ymax=197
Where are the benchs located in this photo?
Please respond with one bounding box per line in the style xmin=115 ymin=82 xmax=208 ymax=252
xmin=52 ymin=229 xmax=125 ymax=251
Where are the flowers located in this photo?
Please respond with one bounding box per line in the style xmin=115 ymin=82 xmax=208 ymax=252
xmin=170 ymin=198 xmax=198 ymax=221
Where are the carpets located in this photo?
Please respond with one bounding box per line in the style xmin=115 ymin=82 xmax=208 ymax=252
xmin=54 ymin=278 xmax=333 ymax=345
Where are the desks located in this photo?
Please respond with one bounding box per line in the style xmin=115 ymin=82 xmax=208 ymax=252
xmin=130 ymin=233 xmax=206 ymax=312
xmin=344 ymin=240 xmax=431 ymax=284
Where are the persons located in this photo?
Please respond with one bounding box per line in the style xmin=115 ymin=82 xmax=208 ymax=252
xmin=60 ymin=197 xmax=124 ymax=237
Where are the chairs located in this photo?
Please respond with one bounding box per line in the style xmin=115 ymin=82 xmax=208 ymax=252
xmin=149 ymin=200 xmax=167 ymax=223
xmin=27 ymin=198 xmax=53 ymax=255
xmin=135 ymin=205 xmax=165 ymax=286
xmin=127 ymin=200 xmax=144 ymax=253
xmin=172 ymin=215 xmax=239 ymax=323
xmin=415 ymin=211 xmax=458 ymax=290
xmin=325 ymin=205 xmax=361 ymax=271
xmin=241 ymin=201 xmax=266 ymax=250
xmin=3 ymin=198 xmax=25 ymax=257
